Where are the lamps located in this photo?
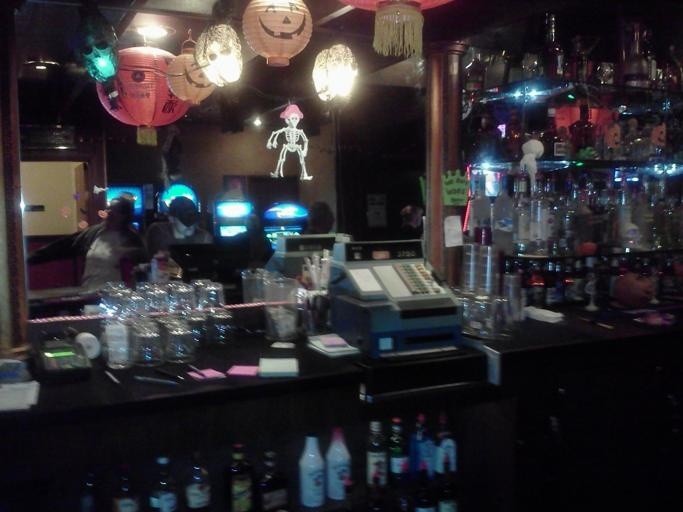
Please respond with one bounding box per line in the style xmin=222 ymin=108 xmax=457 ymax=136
xmin=311 ymin=45 xmax=357 ymax=104
xmin=84 ymin=34 xmax=117 ymax=81
xmin=193 ymin=23 xmax=243 ymax=88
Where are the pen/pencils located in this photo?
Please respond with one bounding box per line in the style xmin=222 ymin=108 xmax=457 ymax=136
xmin=185 ymin=362 xmax=208 ymax=378
xmin=134 ymin=376 xmax=178 ymax=385
xmin=104 ymin=369 xmax=121 ymax=385
xmin=152 ymin=366 xmax=183 ymax=380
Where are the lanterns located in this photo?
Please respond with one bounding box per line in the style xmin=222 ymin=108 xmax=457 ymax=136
xmin=165 ymin=54 xmax=213 ymax=106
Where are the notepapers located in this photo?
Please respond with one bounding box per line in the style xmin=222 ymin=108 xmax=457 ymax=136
xmin=187 ymin=369 xmax=222 ymax=381
xmin=306 ymin=333 xmax=360 ymax=357
xmin=227 ymin=365 xmax=259 ymax=377
xmin=259 ymin=357 xmax=298 ymax=377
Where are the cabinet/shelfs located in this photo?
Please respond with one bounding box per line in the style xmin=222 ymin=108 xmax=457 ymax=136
xmin=3 ymin=302 xmax=682 ymax=511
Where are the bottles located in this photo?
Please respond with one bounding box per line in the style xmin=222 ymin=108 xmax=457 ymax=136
xmin=541 ymin=8 xmax=577 ymax=80
xmin=368 ymin=485 xmax=457 ymax=512
xmin=113 ymin=460 xmax=142 ymax=512
xmin=261 ymin=450 xmax=289 ymax=512
xmin=491 ymin=167 xmax=683 ymax=322
xmin=298 ymin=429 xmax=327 ymax=508
xmin=437 ymin=406 xmax=457 ymax=478
xmin=148 ymin=454 xmax=179 ymax=511
xmin=390 ymin=414 xmax=413 ymax=487
xmin=365 ymin=420 xmax=388 ymax=492
xmin=226 ymin=441 xmax=255 ymax=511
xmin=327 ymin=425 xmax=353 ymax=501
xmin=181 ymin=448 xmax=213 ymax=512
xmin=77 ymin=463 xmax=99 ymax=508
xmin=412 ymin=410 xmax=437 ymax=481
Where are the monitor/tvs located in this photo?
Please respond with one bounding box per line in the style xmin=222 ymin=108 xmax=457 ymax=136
xmin=218 ymin=225 xmax=247 ymax=238
xmin=267 ymin=230 xmax=300 ymax=251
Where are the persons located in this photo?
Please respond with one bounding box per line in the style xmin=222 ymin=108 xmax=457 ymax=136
xmin=305 ymin=202 xmax=335 ymax=233
xmin=400 ymin=205 xmax=423 ymax=228
xmin=145 ymin=198 xmax=219 ymax=248
xmin=28 ymin=194 xmax=145 ymax=286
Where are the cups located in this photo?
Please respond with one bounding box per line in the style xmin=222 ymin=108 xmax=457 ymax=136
xmin=263 ymin=276 xmax=298 ymax=339
xmin=207 ymin=309 xmax=233 ymax=346
xmin=198 ymin=281 xmax=226 ymax=308
xmin=472 ymin=296 xmax=492 ymax=328
xmin=100 ymin=280 xmax=169 ymax=316
xmin=462 ymin=242 xmax=523 ymax=323
xmin=242 ymin=268 xmax=269 ymax=304
xmin=191 ymin=278 xmax=210 ymax=293
xmin=493 ymin=298 xmax=511 ymax=333
xmin=166 ymin=329 xmax=200 ymax=364
xmin=182 ymin=314 xmax=207 ymax=347
xmin=103 ymin=321 xmax=134 ymax=370
xmin=168 ymin=282 xmax=196 ymax=314
xmin=132 ymin=321 xmax=162 ymax=370
xmin=458 ymin=297 xmax=470 ymax=321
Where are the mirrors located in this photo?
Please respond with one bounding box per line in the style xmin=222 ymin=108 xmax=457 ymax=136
xmin=1 ymin=0 xmax=442 ymax=354
